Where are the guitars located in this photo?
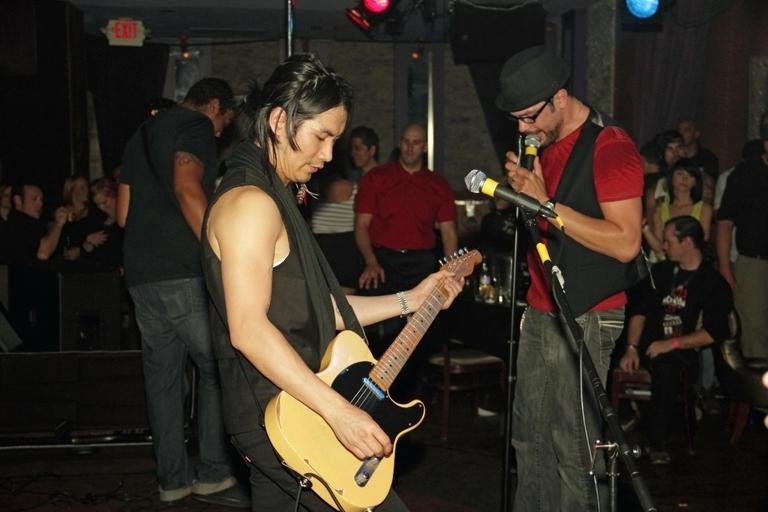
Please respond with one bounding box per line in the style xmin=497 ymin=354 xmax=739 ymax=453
xmin=263 ymin=248 xmax=481 ymax=512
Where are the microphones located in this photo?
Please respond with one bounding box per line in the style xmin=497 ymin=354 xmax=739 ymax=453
xmin=465 ymin=169 xmax=559 ymax=222
xmin=522 ymin=136 xmax=540 ymax=167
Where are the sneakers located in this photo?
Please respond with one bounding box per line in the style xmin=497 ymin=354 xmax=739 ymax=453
xmin=188 ymin=479 xmax=252 ymax=509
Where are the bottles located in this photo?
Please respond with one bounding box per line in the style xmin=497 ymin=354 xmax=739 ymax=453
xmin=478 ymin=254 xmax=500 ymax=305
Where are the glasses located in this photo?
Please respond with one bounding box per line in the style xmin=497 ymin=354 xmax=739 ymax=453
xmin=508 ymin=101 xmax=549 ymax=123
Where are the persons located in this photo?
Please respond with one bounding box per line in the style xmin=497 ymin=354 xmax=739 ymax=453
xmin=490 ymin=44 xmax=646 ymax=512
xmin=197 ymin=51 xmax=463 ymax=512
xmin=603 ymin=215 xmax=734 ymax=467
xmin=117 ymin=77 xmax=241 ymax=501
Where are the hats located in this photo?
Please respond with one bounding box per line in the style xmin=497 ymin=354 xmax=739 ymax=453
xmin=495 ymin=44 xmax=575 ymax=115
